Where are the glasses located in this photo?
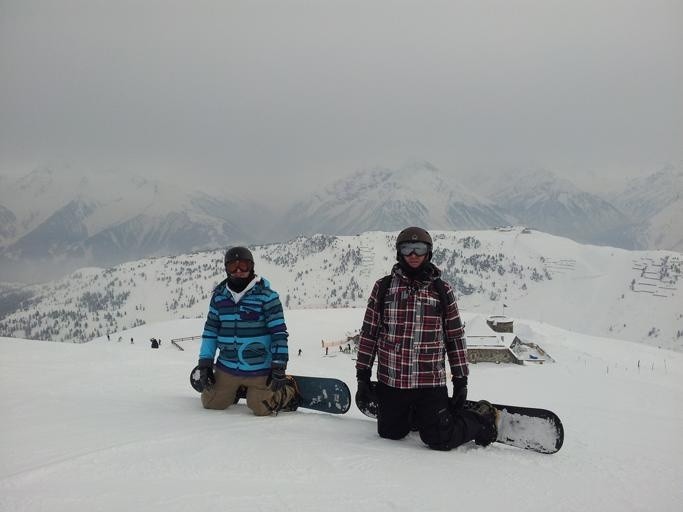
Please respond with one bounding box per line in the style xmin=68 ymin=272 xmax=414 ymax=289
xmin=224 ymin=260 xmax=251 ymax=272
xmin=399 ymin=242 xmax=429 ymax=256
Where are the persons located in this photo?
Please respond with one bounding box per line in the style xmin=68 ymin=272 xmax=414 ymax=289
xmin=198 ymin=246 xmax=303 ymax=416
xmin=353 ymin=226 xmax=499 ymax=452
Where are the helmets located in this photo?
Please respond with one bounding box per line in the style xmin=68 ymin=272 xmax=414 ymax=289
xmin=396 ymin=227 xmax=432 ymax=248
xmin=224 ymin=247 xmax=254 ymax=265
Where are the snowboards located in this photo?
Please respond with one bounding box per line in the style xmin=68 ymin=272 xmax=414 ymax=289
xmin=355 ymin=381 xmax=564 ymax=454
xmin=190 ymin=365 xmax=351 ymax=414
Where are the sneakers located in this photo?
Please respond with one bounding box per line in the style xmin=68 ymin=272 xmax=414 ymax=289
xmin=472 ymin=400 xmax=497 ymax=445
xmin=284 ymin=374 xmax=300 ymax=411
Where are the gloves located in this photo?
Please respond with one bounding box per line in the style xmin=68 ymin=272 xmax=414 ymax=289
xmin=266 ymin=361 xmax=287 ymax=391
xmin=357 ymin=379 xmax=377 ymax=402
xmin=198 ymin=358 xmax=215 ymax=388
xmin=450 ymin=376 xmax=467 ymax=410
